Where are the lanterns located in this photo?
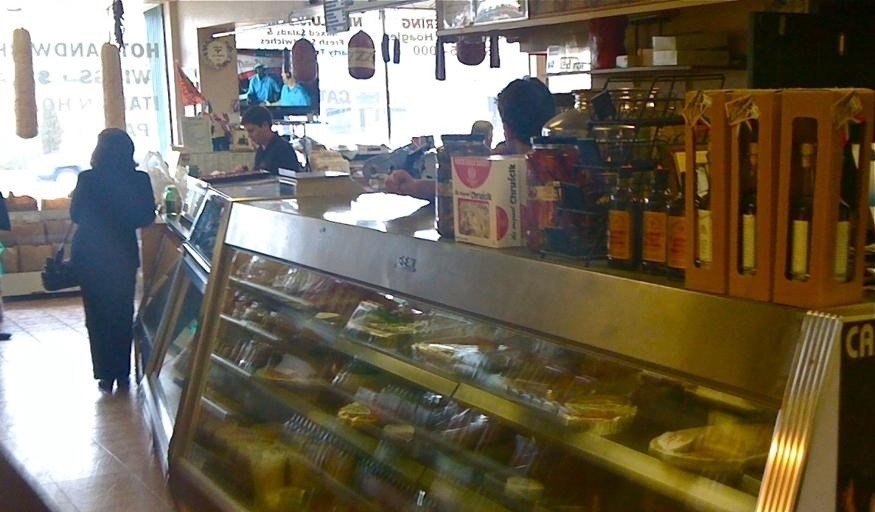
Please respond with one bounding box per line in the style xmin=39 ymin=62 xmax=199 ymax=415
xmin=347 ymin=29 xmax=377 ymax=80
xmin=455 ymin=36 xmax=487 ymax=65
xmin=292 ymin=38 xmax=318 ymax=84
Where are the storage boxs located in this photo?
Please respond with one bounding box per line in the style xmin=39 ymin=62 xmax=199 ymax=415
xmin=450 ymin=155 xmax=531 ymax=248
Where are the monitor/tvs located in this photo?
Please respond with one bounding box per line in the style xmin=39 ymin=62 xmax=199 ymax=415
xmin=236 ymin=48 xmax=320 ymax=116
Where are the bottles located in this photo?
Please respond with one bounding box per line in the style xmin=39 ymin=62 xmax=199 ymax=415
xmin=164 ymin=186 xmax=179 ymax=218
xmin=606 ymin=141 xmax=852 ymax=288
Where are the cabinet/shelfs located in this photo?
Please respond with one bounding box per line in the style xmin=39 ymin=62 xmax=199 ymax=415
xmin=539 ymin=74 xmax=727 ymax=268
xmin=131 ymin=174 xmax=875 ymax=512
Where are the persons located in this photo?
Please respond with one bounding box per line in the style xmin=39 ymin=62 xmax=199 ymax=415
xmin=471 ymin=119 xmax=494 ymax=150
xmin=70 ymin=128 xmax=157 ymax=392
xmin=259 ymin=70 xmax=312 ymax=107
xmin=245 ymin=61 xmax=279 ymax=105
xmin=232 ymin=104 xmax=303 ymax=173
xmin=0 ymin=189 xmax=14 ymax=339
xmin=378 ymin=74 xmax=557 ymax=202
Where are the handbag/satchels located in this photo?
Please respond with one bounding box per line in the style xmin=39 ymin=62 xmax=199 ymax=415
xmin=41 ymin=220 xmax=80 ymax=292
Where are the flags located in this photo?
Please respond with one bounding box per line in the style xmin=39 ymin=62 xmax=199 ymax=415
xmin=174 ymin=62 xmax=208 ymax=106
